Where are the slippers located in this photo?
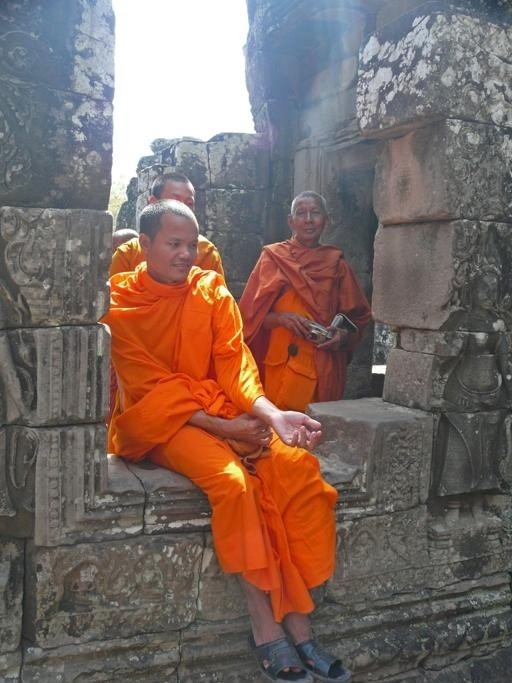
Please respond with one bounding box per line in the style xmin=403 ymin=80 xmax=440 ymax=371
xmin=248 ymin=632 xmax=314 ymax=683
xmin=295 ymin=639 xmax=352 ymax=683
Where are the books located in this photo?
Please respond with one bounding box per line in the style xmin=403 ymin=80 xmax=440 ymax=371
xmin=324 ymin=312 xmax=361 ymax=340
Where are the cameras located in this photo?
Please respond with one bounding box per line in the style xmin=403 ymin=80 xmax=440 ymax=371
xmin=304 ymin=319 xmax=330 ymax=346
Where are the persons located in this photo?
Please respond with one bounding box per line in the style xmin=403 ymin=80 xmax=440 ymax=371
xmin=112 ymin=224 xmax=139 ymax=256
xmin=97 ymin=198 xmax=357 ymax=682
xmin=236 ymin=187 xmax=371 ymax=413
xmin=110 ymin=170 xmax=225 ymax=281
